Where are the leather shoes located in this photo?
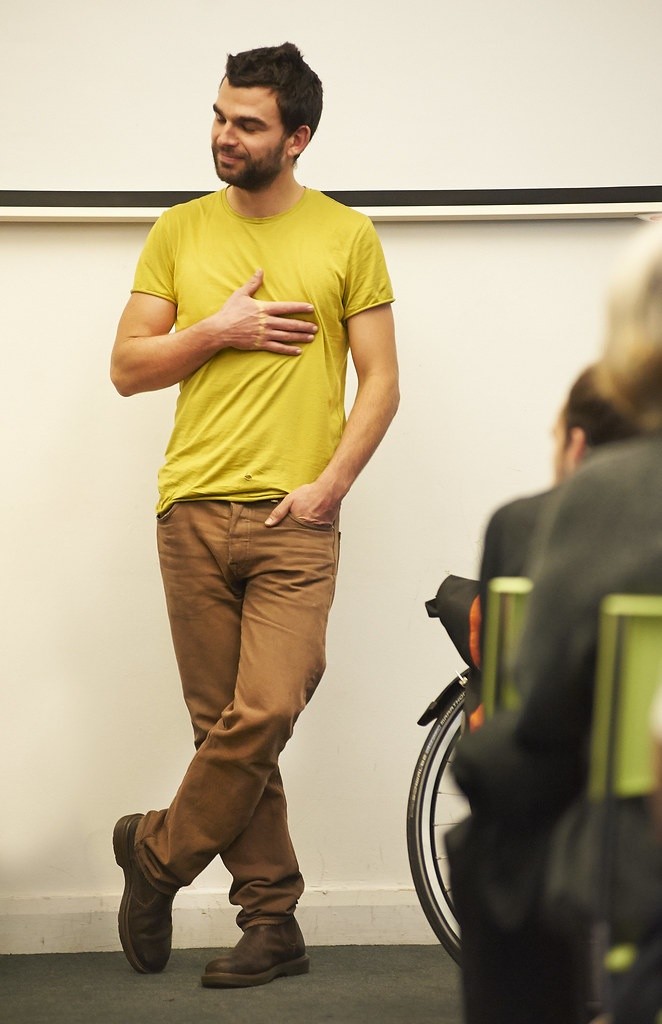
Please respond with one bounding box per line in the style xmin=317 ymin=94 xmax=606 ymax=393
xmin=208 ymin=917 xmax=314 ymax=981
xmin=112 ymin=813 xmax=174 ymax=970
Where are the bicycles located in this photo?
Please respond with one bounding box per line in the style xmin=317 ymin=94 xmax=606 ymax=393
xmin=399 ymin=667 xmax=477 ymax=977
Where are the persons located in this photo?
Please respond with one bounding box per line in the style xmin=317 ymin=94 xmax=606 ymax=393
xmin=110 ymin=42 xmax=400 ymax=988
xmin=443 ymin=217 xmax=662 ymax=1024
